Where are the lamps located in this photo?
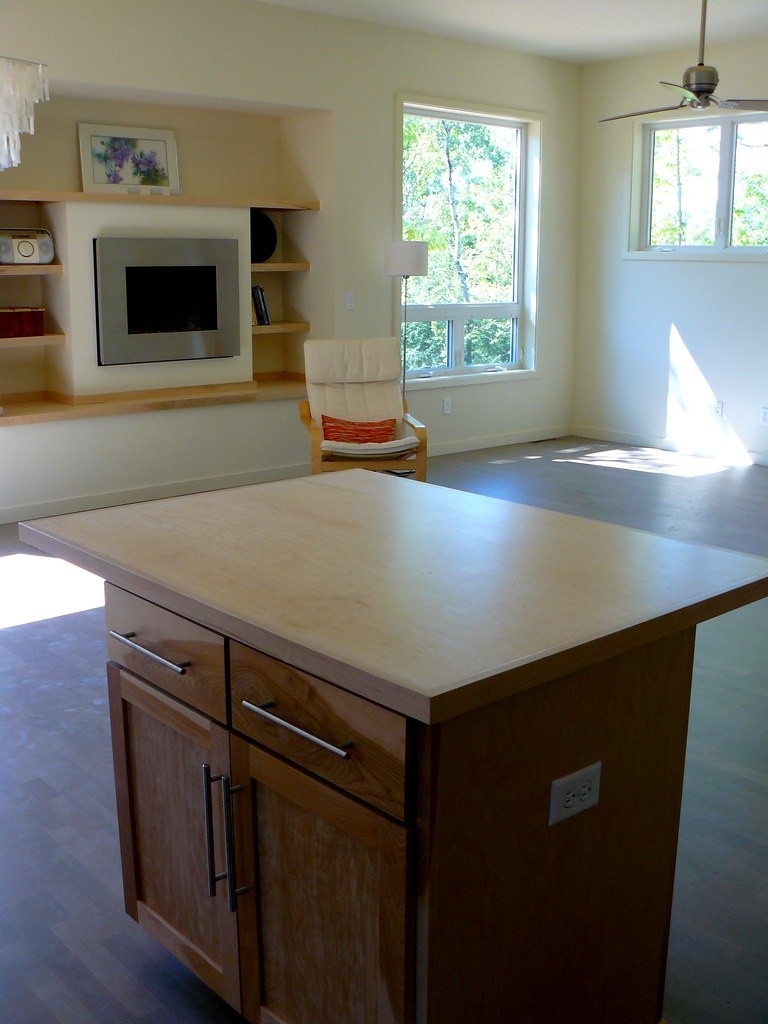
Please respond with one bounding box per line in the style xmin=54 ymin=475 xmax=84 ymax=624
xmin=384 ymin=240 xmax=428 ymax=474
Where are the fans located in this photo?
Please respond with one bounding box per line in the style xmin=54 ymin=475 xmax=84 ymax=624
xmin=597 ymin=0 xmax=768 ymax=123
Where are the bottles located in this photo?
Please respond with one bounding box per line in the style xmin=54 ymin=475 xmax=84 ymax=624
xmin=251 ymin=286 xmax=270 ymax=325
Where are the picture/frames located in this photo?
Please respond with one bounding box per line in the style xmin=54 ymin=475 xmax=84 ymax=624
xmin=76 ymin=120 xmax=180 ymax=195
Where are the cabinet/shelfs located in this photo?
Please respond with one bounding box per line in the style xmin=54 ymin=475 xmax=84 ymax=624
xmin=16 ymin=468 xmax=768 ymax=1024
xmin=0 ymin=143 xmax=316 ymax=390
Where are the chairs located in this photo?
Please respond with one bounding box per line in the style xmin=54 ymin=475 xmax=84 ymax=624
xmin=298 ymin=337 xmax=426 ymax=482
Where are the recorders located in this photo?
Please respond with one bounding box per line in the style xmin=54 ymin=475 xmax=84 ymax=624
xmin=0 ymin=228 xmax=55 ymax=265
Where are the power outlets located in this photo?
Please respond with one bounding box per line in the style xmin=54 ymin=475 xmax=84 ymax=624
xmin=345 ymin=291 xmax=354 ymax=311
xmin=443 ymin=398 xmax=452 ymax=415
